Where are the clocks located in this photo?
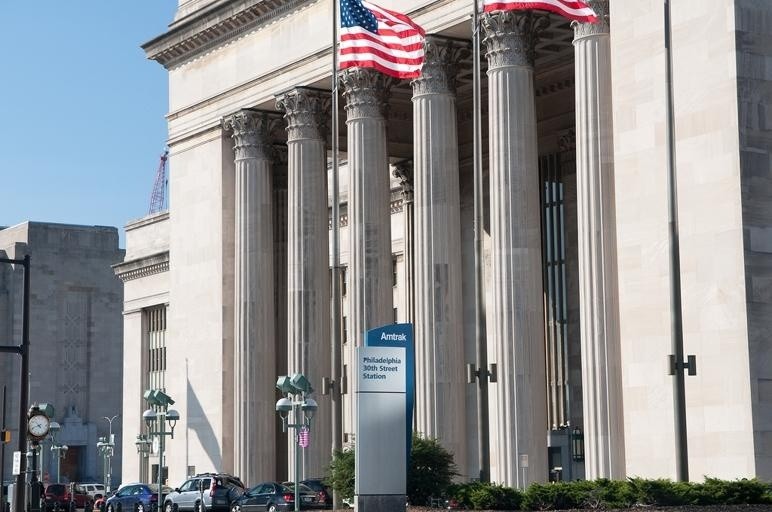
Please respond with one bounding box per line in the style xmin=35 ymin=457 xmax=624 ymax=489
xmin=26 ymin=405 xmax=50 ymax=512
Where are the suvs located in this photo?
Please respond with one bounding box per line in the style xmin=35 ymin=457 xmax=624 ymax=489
xmin=42 ymin=483 xmax=94 ymax=509
xmin=76 ymin=481 xmax=106 ymax=500
xmin=161 ymin=471 xmax=246 ymax=511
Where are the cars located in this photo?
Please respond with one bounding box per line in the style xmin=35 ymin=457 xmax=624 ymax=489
xmin=104 ymin=482 xmax=175 ymax=512
xmin=343 ymin=497 xmax=354 ymax=509
xmin=229 ymin=479 xmax=320 ymax=512
xmin=94 ymin=495 xmax=108 ymax=509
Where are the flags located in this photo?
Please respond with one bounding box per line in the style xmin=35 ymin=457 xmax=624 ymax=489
xmin=482 ymin=0 xmax=601 ymax=24
xmin=336 ymin=0 xmax=425 ymax=79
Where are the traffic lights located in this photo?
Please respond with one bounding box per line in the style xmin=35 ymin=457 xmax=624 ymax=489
xmin=1 ymin=431 xmax=6 ymax=441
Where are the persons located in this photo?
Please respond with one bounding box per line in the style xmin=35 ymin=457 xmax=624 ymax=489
xmin=39 ymin=494 xmax=46 ymax=512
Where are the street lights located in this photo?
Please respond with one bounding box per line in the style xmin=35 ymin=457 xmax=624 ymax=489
xmin=102 ymin=414 xmax=118 ymax=434
xmin=37 ymin=400 xmax=63 ymax=482
xmin=49 ymin=444 xmax=70 ymax=484
xmin=96 ymin=435 xmax=115 ymax=487
xmin=136 ymin=434 xmax=152 ymax=486
xmin=141 ymin=388 xmax=182 ymax=512
xmin=274 ymin=373 xmax=321 ymax=512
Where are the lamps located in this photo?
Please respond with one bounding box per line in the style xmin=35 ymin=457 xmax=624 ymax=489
xmin=571 ymin=426 xmax=583 ymax=463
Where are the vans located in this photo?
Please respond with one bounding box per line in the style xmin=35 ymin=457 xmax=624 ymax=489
xmin=298 ymin=477 xmax=334 ymax=508
